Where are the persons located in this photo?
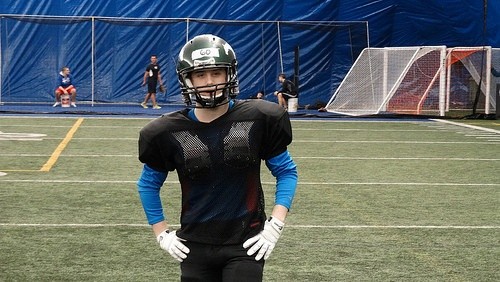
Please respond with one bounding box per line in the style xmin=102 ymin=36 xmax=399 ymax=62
xmin=52 ymin=66 xmax=77 ymax=107
xmin=136 ymin=34 xmax=298 ymax=282
xmin=274 ymin=74 xmax=298 ymax=110
xmin=140 ymin=54 xmax=165 ymax=109
xmin=249 ymin=90 xmax=264 ymax=99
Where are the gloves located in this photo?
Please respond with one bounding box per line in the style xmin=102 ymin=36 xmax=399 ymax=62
xmin=243 ymin=215 xmax=285 ymax=261
xmin=157 ymin=229 xmax=190 ymax=262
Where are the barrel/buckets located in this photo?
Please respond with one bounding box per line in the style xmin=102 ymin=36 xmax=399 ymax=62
xmin=60 ymin=95 xmax=71 ymax=108
xmin=287 ymin=98 xmax=298 ymax=113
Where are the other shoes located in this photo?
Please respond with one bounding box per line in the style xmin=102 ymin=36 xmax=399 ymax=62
xmin=141 ymin=103 xmax=148 ymax=108
xmin=53 ymin=103 xmax=60 ymax=107
xmin=71 ymin=103 xmax=76 ymax=107
xmin=153 ymin=105 xmax=161 ymax=109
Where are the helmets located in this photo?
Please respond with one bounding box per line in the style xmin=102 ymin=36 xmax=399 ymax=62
xmin=176 ymin=34 xmax=239 ymax=108
